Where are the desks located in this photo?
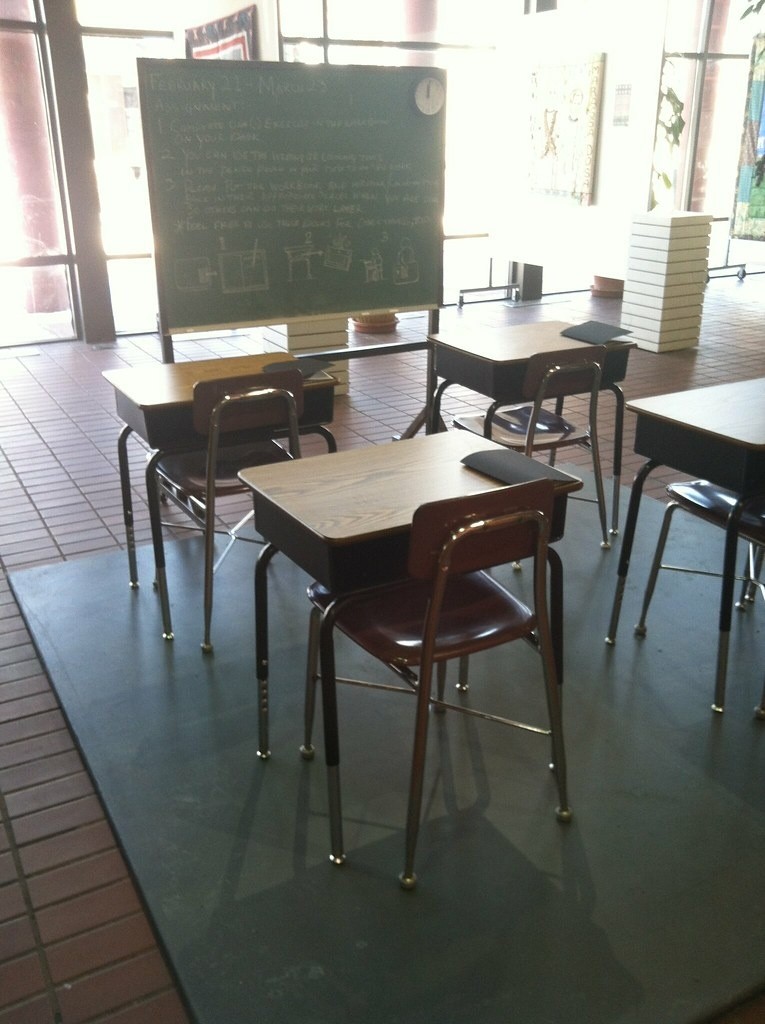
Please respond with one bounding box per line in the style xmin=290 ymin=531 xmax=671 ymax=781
xmin=237 ymin=430 xmax=583 ymax=866
xmin=606 ymin=374 xmax=765 ymax=715
xmin=427 ymin=321 xmax=638 ymax=536
xmin=102 ymin=352 xmax=339 ymax=643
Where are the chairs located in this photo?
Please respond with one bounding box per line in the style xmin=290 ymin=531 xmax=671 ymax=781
xmin=633 ymin=478 xmax=765 ymax=719
xmin=146 ymin=367 xmax=305 ymax=655
xmin=452 ymin=344 xmax=612 ymax=570
xmin=300 ymin=476 xmax=572 ymax=891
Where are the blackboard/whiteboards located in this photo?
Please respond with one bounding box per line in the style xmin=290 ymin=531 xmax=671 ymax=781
xmin=138 ymin=57 xmax=444 ymax=334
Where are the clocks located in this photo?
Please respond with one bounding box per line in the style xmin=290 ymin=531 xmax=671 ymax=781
xmin=409 ymin=73 xmax=445 ymax=116
